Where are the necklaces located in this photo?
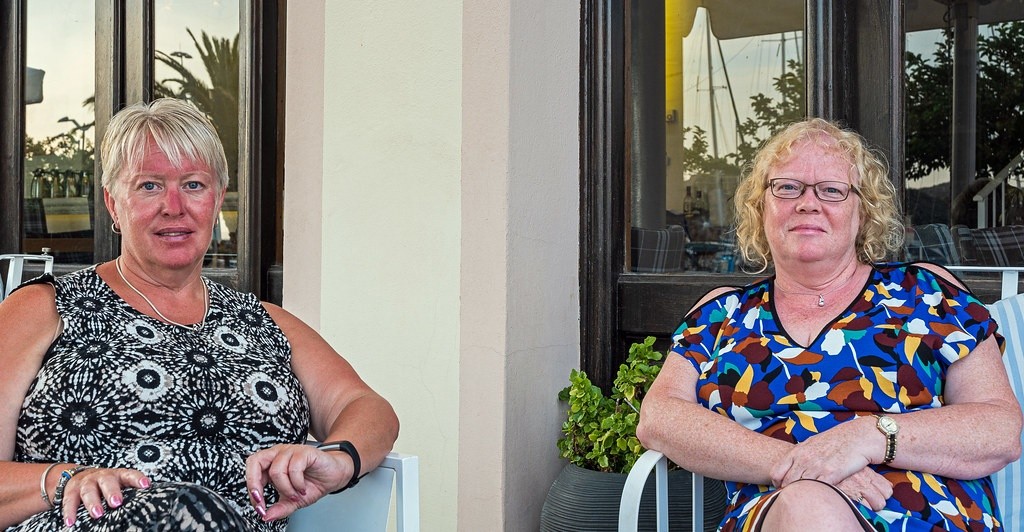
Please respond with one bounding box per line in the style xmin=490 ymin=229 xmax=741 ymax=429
xmin=772 ymin=277 xmax=856 ymax=306
xmin=112 ymin=256 xmax=208 ymax=335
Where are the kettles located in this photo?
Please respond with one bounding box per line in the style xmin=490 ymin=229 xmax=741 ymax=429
xmin=46 ymin=170 xmax=66 ymax=197
xmin=77 ymin=170 xmax=95 ymax=197
xmin=61 ymin=170 xmax=82 ymax=196
xmin=31 ymin=168 xmax=49 ymax=197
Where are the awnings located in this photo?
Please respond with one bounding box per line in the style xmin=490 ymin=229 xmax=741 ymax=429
xmin=665 ymin=0 xmax=1024 ymax=40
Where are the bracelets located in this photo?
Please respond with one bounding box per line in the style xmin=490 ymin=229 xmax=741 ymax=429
xmin=39 ymin=461 xmax=95 ymax=509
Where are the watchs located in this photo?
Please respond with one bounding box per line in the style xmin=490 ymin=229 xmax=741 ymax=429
xmin=312 ymin=440 xmax=361 ymax=495
xmin=872 ymin=414 xmax=900 ymax=464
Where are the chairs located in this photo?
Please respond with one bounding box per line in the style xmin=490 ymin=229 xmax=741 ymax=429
xmin=968 ymin=226 xmax=1024 ymax=277
xmin=899 ymin=224 xmax=961 ymax=269
xmin=283 ymin=444 xmax=421 ymax=531
xmin=21 ymin=200 xmax=48 ymax=238
xmin=619 ymin=268 xmax=1018 ymax=532
xmin=631 ymin=223 xmax=682 ymax=272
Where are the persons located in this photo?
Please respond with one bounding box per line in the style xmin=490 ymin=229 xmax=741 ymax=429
xmin=0 ymin=98 xmax=400 ymax=531
xmin=635 ymin=117 xmax=1024 ymax=532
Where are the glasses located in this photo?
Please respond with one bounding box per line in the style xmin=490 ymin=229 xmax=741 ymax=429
xmin=763 ymin=177 xmax=858 ymax=203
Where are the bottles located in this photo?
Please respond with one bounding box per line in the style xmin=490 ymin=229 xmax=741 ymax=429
xmin=683 ymin=185 xmax=693 ymax=213
xmin=721 ymin=253 xmax=736 ymax=273
xmin=694 ymin=190 xmax=706 ymax=210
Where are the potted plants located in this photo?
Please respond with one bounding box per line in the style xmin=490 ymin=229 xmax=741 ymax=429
xmin=538 ymin=336 xmax=727 ymax=532
